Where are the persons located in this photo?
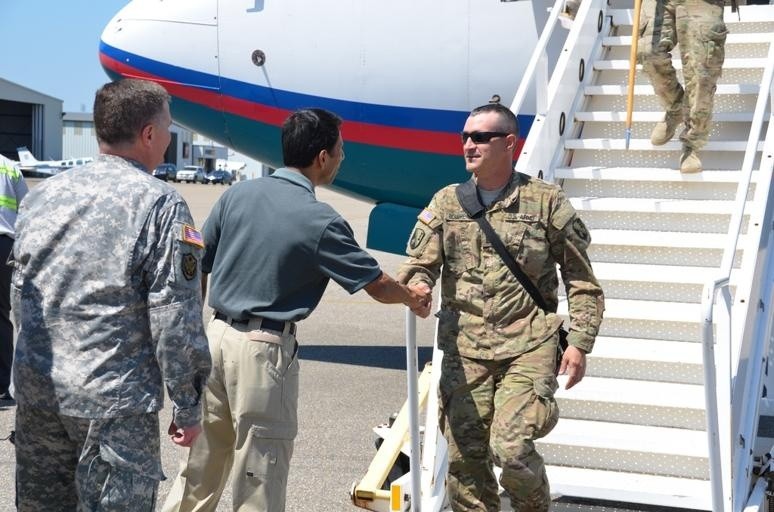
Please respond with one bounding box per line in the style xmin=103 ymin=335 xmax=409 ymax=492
xmin=151 ymin=105 xmax=433 ymax=511
xmin=5 ymin=75 xmax=215 ymax=512
xmin=0 ymin=148 xmax=30 ymax=409
xmin=631 ymin=0 xmax=729 ymax=175
xmin=394 ymin=88 xmax=610 ymax=510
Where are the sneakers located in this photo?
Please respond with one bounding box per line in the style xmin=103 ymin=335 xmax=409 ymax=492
xmin=0 ymin=392 xmax=13 ymax=407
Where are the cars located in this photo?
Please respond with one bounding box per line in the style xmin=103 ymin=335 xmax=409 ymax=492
xmin=176 ymin=165 xmax=206 ymax=184
xmin=203 ymin=170 xmax=233 ymax=185
xmin=152 ymin=162 xmax=178 ymax=182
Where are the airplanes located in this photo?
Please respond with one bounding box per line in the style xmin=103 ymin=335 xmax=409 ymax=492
xmin=14 ymin=145 xmax=94 ymax=176
xmin=97 ymin=2 xmax=769 ymax=512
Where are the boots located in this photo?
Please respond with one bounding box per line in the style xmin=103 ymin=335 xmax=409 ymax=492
xmin=652 ymin=109 xmax=683 ymax=145
xmin=679 ymin=143 xmax=701 ymax=173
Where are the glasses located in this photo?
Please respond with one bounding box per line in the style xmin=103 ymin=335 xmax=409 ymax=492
xmin=462 ymin=132 xmax=508 ymax=143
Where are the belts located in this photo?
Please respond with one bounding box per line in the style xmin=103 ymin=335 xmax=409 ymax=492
xmin=216 ymin=314 xmax=295 ymax=334
